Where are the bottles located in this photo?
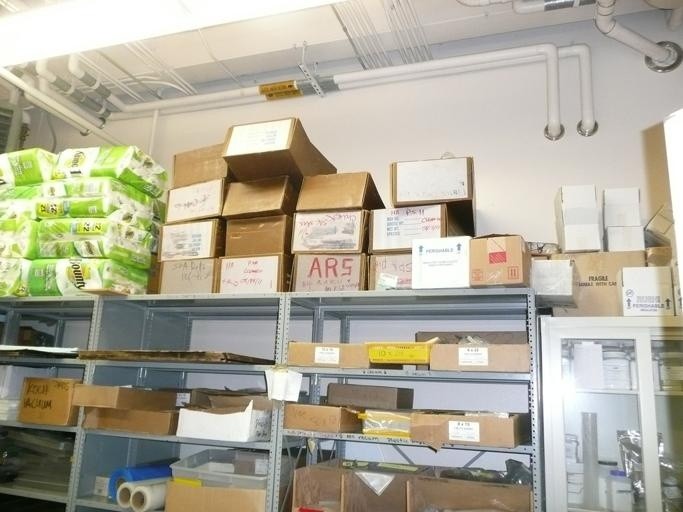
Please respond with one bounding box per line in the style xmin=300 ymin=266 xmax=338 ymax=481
xmin=596 ymin=459 xmax=633 ymax=512
xmin=602 ymin=351 xmax=683 ymax=392
xmin=580 ymin=411 xmax=601 ymax=508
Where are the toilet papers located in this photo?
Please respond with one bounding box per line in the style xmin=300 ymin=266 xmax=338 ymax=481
xmin=107 ymin=455 xmax=181 ymax=511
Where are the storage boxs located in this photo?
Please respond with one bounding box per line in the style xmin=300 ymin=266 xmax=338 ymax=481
xmin=12 ymin=329 xmax=537 ymax=511
xmin=150 ymin=111 xmax=682 ymax=317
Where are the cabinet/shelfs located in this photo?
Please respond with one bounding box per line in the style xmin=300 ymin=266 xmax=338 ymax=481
xmin=531 ymin=311 xmax=682 ymax=511
xmin=66 ymin=284 xmax=289 ymax=510
xmin=0 ymin=293 xmax=193 ymax=511
xmin=269 ymin=285 xmax=545 ymax=511
